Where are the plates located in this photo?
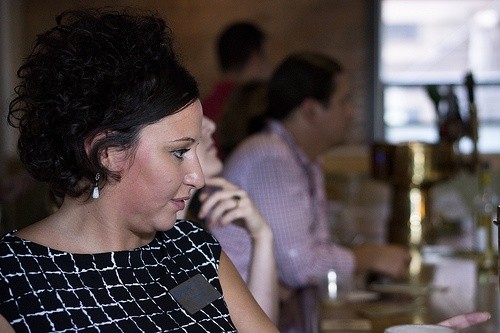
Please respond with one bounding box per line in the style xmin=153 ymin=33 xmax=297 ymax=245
xmin=385 ymin=324 xmax=455 ymax=333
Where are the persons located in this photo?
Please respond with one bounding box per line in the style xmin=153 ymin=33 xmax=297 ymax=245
xmin=205 ymin=50 xmax=415 ymax=331
xmin=198 ymin=19 xmax=275 ymax=161
xmin=197 ymin=114 xmax=277 ymax=324
xmin=0 ymin=3 xmax=277 ymax=333
